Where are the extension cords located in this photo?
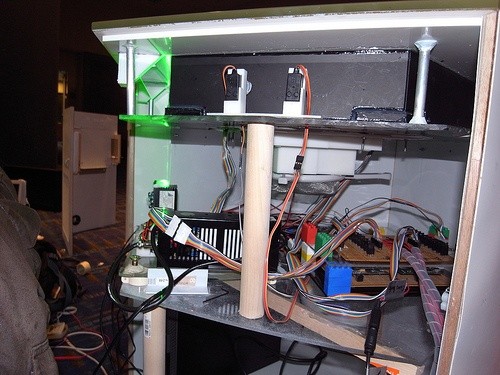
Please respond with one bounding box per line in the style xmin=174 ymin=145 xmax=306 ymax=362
xmin=47 ymin=322 xmax=68 ymax=339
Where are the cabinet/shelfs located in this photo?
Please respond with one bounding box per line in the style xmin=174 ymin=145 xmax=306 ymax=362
xmin=90 ymin=0 xmax=500 ymax=375
